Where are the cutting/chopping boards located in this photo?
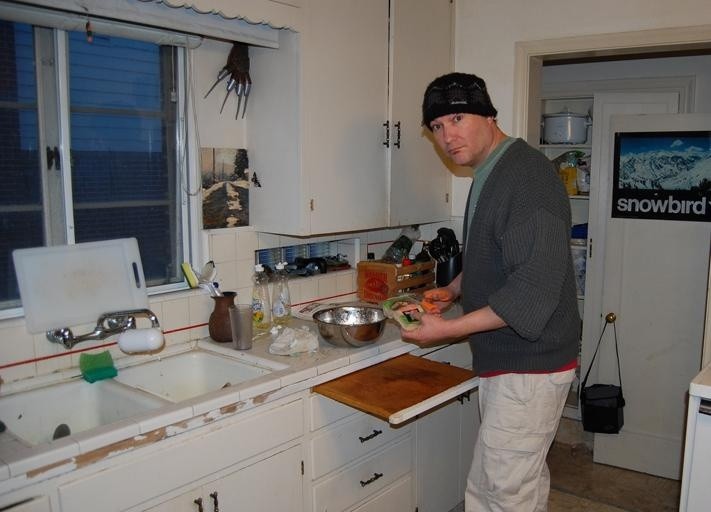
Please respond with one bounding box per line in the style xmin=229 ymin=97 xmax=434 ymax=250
xmin=310 ymin=353 xmax=486 ymax=431
xmin=11 ymin=237 xmax=152 ymax=335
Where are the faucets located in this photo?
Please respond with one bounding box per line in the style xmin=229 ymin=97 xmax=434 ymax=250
xmin=48 ymin=327 xmax=80 ymax=350
xmin=95 ymin=308 xmax=161 ymax=341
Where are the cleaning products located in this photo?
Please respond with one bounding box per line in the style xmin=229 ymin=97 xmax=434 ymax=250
xmin=271 ymin=259 xmax=293 ymax=327
xmin=249 ymin=263 xmax=273 ymax=330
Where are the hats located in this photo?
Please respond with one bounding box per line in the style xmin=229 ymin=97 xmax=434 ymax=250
xmin=420 ymin=71 xmax=496 ymax=123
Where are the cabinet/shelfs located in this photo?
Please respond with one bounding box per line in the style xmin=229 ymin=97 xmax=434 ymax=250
xmin=355 ymin=256 xmax=441 ymax=304
xmin=309 ymin=353 xmax=481 ymax=512
xmin=540 ymin=90 xmax=681 ymax=422
xmin=249 ymin=1 xmax=457 ymax=237
xmin=419 ymin=341 xmax=501 ymax=512
xmin=55 ymin=399 xmax=304 ymax=512
xmin=0 ymin=497 xmax=50 ymax=512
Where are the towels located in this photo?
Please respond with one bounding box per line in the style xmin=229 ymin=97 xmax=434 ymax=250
xmin=269 ymin=324 xmax=319 ymax=356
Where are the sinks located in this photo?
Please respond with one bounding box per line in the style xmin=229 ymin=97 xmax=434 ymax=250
xmin=1 ymin=377 xmax=161 ymax=447
xmin=115 ymin=340 xmax=279 ymax=402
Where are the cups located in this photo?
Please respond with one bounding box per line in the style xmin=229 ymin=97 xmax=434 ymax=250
xmin=226 ymin=303 xmax=255 ymax=352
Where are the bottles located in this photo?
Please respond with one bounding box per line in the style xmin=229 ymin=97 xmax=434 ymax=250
xmin=273 ymin=263 xmax=292 ymax=325
xmin=251 ymin=265 xmax=271 ymax=334
xmin=382 ymin=222 xmax=421 ymax=264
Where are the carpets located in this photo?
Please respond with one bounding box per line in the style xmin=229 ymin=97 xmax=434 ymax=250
xmin=544 ymin=437 xmax=680 ymax=512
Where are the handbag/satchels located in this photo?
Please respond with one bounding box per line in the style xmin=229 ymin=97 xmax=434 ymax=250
xmin=581 ymin=316 xmax=623 ymax=437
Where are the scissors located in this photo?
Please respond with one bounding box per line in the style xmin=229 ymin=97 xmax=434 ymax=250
xmin=409 ymin=288 xmax=449 ymax=316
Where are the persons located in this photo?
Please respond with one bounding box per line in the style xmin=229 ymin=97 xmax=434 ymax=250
xmin=402 ymin=73 xmax=580 ymax=512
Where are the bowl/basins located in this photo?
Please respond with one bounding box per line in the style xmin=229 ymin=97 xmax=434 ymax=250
xmin=310 ymin=304 xmax=390 ymax=350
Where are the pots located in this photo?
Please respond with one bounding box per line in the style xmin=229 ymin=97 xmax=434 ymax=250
xmin=540 ymin=106 xmax=595 ymax=147
